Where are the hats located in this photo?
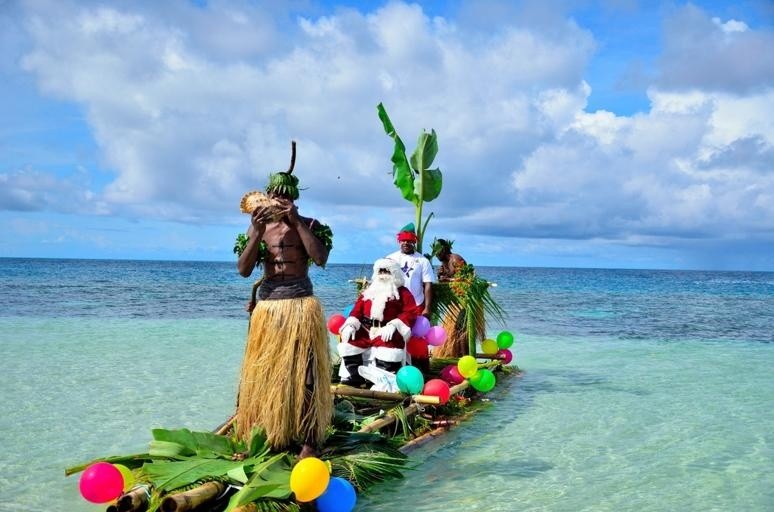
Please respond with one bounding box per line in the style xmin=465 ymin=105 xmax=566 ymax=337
xmin=371 ymin=258 xmax=406 ymax=286
xmin=397 ymin=222 xmax=417 ymax=243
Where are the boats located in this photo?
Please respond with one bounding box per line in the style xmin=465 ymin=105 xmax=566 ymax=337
xmin=102 ymin=275 xmax=524 ymax=512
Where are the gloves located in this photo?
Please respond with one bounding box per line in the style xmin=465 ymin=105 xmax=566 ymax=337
xmin=341 ymin=326 xmax=355 ymax=344
xmin=381 ymin=324 xmax=395 ymax=342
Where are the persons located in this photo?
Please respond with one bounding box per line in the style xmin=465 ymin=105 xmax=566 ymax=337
xmin=435 ymin=239 xmax=485 ymax=340
xmin=231 ymin=184 xmax=332 ymax=463
xmin=385 ymin=233 xmax=434 ymax=372
xmin=337 ymin=258 xmax=417 ymax=389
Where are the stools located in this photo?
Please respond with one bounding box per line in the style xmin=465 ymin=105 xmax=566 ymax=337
xmin=340 ymin=335 xmax=408 ymax=382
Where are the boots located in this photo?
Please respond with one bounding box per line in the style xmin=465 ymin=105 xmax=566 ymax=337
xmin=373 ymin=347 xmax=404 ymax=387
xmin=337 ymin=344 xmax=365 ymax=386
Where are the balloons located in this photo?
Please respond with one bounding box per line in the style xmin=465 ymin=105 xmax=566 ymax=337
xmin=328 ymin=303 xmax=355 ymax=344
xmin=396 ymin=316 xmax=513 ymax=409
xmin=106 ymin=463 xmax=134 ymax=504
xmin=290 ymin=457 xmax=330 ymax=503
xmin=315 ymin=477 xmax=357 ymax=512
xmin=80 ymin=462 xmax=124 ymax=504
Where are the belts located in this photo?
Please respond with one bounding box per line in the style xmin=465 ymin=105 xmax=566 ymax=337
xmin=361 ymin=317 xmax=390 ymax=328
